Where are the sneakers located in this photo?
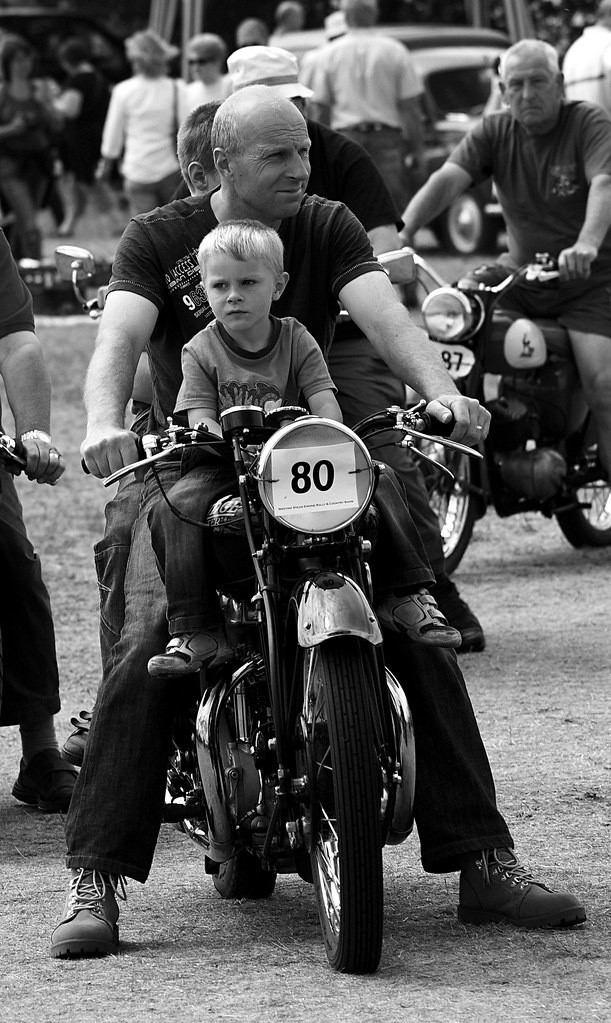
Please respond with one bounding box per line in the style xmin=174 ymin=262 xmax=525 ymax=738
xmin=62 ymin=710 xmax=95 ymax=768
xmin=148 ymin=631 xmax=234 ymax=676
xmin=12 ymin=750 xmax=79 ymax=812
xmin=457 ymin=848 xmax=587 ymax=929
xmin=432 ymin=575 xmax=485 ymax=652
xmin=47 ymin=864 xmax=123 ymax=957
xmin=377 ymin=589 xmax=460 ymax=645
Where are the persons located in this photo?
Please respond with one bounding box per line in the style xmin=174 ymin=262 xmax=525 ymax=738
xmin=145 ymin=217 xmax=466 ymax=675
xmin=47 ymin=86 xmax=590 ymax=956
xmin=398 ymin=34 xmax=611 ymax=485
xmin=0 ymin=224 xmax=82 ymax=812
xmin=2 ymin=3 xmax=611 ymax=311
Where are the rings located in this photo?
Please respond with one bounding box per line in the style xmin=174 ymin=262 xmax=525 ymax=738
xmin=49 ymin=447 xmax=59 ymax=459
xmin=476 ymin=425 xmax=484 ymax=431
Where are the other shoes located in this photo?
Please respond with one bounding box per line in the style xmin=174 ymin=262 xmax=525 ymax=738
xmin=45 ymin=228 xmax=72 ymax=237
xmin=406 ymin=284 xmax=419 ymax=311
xmin=113 ymin=226 xmax=125 ymax=236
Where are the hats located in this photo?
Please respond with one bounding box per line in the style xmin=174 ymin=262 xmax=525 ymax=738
xmin=227 ymin=45 xmax=312 ymax=106
xmin=122 ymin=30 xmax=180 ymax=61
xmin=325 ymin=11 xmax=350 ymax=39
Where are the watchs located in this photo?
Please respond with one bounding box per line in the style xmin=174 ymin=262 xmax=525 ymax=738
xmin=19 ymin=428 xmax=53 ymax=445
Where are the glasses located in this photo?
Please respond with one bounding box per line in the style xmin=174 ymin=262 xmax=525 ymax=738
xmin=187 ymin=57 xmax=212 ymax=66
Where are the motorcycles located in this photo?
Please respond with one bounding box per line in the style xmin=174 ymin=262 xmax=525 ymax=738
xmin=80 ymin=395 xmax=499 ymax=981
xmin=374 ymin=241 xmax=610 ymax=578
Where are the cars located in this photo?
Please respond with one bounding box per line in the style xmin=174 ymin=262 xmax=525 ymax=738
xmin=235 ymin=11 xmax=562 ymax=254
